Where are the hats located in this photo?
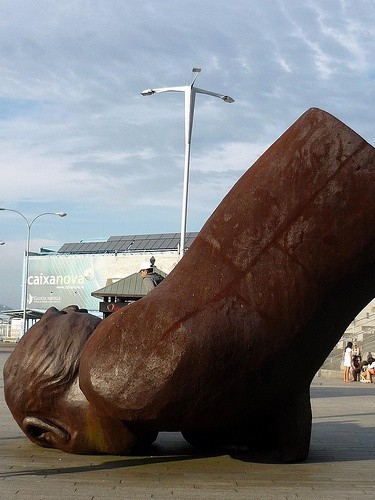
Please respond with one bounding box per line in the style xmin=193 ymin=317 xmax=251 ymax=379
xmin=137 ymin=262 xmax=152 ymax=273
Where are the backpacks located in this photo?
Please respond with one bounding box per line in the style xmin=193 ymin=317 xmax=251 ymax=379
xmin=143 ymin=272 xmax=165 ymax=286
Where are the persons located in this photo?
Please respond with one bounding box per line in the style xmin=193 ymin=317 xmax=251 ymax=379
xmin=3 ymin=107 xmax=375 ymax=465
xmin=137 ymin=262 xmax=166 ymax=293
xmin=352 ymin=357 xmax=375 ymax=383
xmin=341 ymin=342 xmax=353 ymax=382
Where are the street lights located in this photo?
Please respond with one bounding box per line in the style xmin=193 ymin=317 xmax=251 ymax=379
xmin=140 ymin=66 xmax=236 ymax=260
xmin=0 ymin=208 xmax=68 ymax=336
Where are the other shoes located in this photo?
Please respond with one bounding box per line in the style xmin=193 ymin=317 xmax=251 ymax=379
xmin=345 ymin=380 xmax=352 ymax=383
xmin=353 ymin=380 xmax=357 ymax=382
